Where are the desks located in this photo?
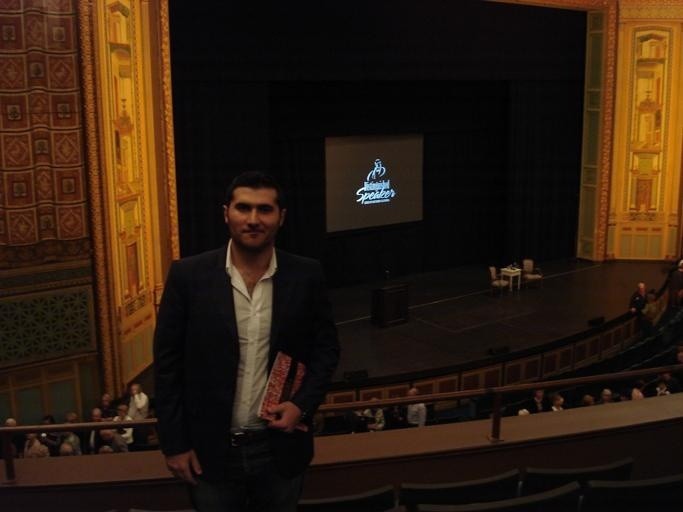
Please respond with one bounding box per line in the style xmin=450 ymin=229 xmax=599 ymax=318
xmin=499 ymin=266 xmax=522 ymax=291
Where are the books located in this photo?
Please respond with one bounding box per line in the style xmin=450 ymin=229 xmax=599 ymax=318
xmin=255 ymin=351 xmax=312 ymax=434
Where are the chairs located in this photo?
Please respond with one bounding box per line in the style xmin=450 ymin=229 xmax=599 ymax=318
xmin=488 ymin=265 xmax=508 ymax=296
xmin=326 ymin=362 xmax=590 ymax=434
xmin=520 ymin=257 xmax=545 ymax=289
xmin=591 ymin=303 xmax=682 ymax=403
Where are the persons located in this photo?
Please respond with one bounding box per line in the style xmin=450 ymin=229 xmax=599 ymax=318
xmin=668 ymin=258 xmax=682 ymax=311
xmin=312 ymin=388 xmax=428 ymax=437
xmin=0 ymin=383 xmax=150 ymax=458
xmin=515 ymin=344 xmax=682 ymax=416
xmin=144 ymin=168 xmax=345 ymax=511
xmin=630 ymin=281 xmax=648 ymax=311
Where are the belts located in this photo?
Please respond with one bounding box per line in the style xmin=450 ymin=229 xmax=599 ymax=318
xmin=228 ymin=431 xmax=269 ymax=447
